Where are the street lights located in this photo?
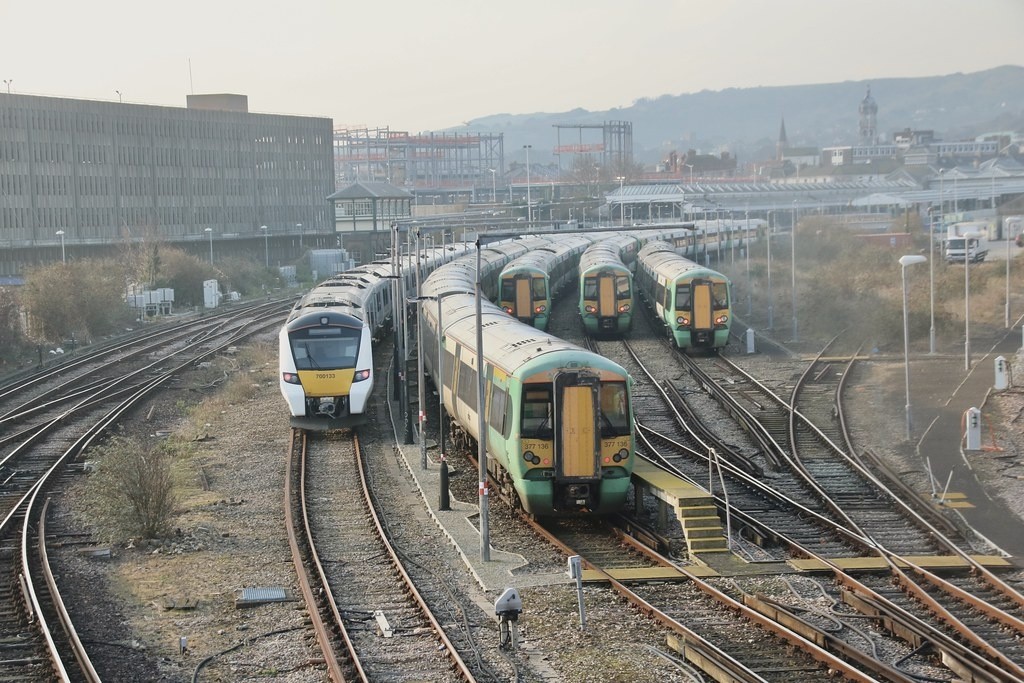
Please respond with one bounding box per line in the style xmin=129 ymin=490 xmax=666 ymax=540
xmin=204 ymin=228 xmax=213 ymax=267
xmin=490 ymin=169 xmax=495 ymax=202
xmin=55 ymin=229 xmax=66 ymax=263
xmin=692 ymin=201 xmax=803 ymax=340
xmin=522 ymin=199 xmax=694 ymax=229
xmin=961 ymin=230 xmax=976 ymax=370
xmin=939 ymin=168 xmax=944 ymax=261
xmin=898 ymin=254 xmax=928 ymax=439
xmin=927 ymin=207 xmax=935 ymax=353
xmin=1005 ymin=215 xmax=1023 ymax=328
xmin=296 ymin=224 xmax=302 ymax=255
xmin=685 ymin=163 xmax=694 ymax=184
xmin=523 ymin=145 xmax=532 ymax=229
xmin=617 ymin=177 xmax=625 ymax=225
xmin=261 ymin=225 xmax=268 ymax=267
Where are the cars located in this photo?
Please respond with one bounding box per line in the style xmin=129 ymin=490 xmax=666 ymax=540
xmin=1015 ymin=232 xmax=1024 ymax=247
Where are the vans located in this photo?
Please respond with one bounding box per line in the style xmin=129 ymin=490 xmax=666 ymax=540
xmin=945 ymin=220 xmax=991 ymax=265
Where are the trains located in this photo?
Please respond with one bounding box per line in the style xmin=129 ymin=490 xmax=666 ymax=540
xmin=497 ymin=231 xmax=623 ymax=333
xmin=276 ymin=232 xmax=543 ymax=434
xmin=636 ymin=240 xmax=734 ymax=350
xmin=576 ymin=218 xmax=768 ymax=333
xmin=420 ymin=231 xmax=653 ymax=522
